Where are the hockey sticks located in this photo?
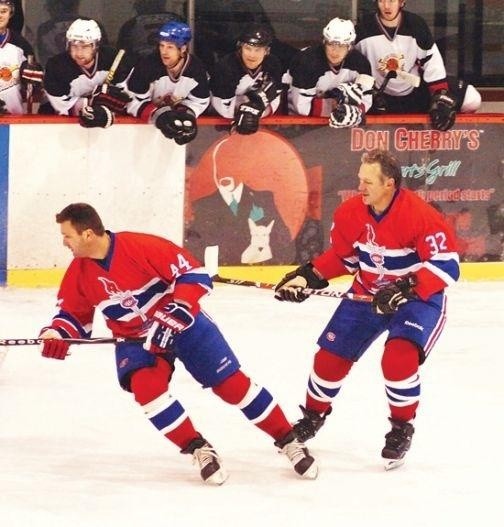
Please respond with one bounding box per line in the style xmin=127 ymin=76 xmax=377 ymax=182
xmin=375 ymin=70 xmax=420 ymax=102
xmin=204 ymin=245 xmax=375 ymax=302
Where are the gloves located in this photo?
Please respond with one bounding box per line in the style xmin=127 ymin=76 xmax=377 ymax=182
xmin=38 ymin=314 xmax=82 ymax=360
xmin=371 ymin=271 xmax=418 ymax=316
xmin=274 ymin=262 xmax=329 ymax=303
xmin=20 ymin=60 xmax=46 ymax=93
xmin=130 ymin=302 xmax=195 ymax=354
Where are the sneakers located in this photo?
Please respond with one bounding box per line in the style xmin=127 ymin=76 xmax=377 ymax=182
xmin=292 ymin=405 xmax=333 ymax=444
xmin=382 ymin=417 xmax=414 ymax=460
xmin=179 ymin=432 xmax=220 ymax=481
xmin=273 ymin=431 xmax=318 ymax=480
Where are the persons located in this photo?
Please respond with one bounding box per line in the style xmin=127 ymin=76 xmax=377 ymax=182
xmin=0 ymin=0 xmax=43 ymax=116
xmin=127 ymin=17 xmax=213 ymax=146
xmin=41 ymin=16 xmax=135 ymax=129
xmin=203 ymin=13 xmax=288 ymax=136
xmin=353 ymin=0 xmax=457 ymax=132
xmin=273 ymin=148 xmax=459 ymax=460
xmin=281 ymin=16 xmax=375 ymax=128
xmin=39 ymin=202 xmax=314 ymax=482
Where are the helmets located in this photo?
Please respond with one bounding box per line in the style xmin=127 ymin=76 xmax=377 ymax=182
xmin=157 ymin=20 xmax=192 ymax=48
xmin=322 ymin=17 xmax=357 ymax=46
xmin=65 ymin=18 xmax=102 ymax=52
xmin=236 ymin=22 xmax=274 ymax=52
